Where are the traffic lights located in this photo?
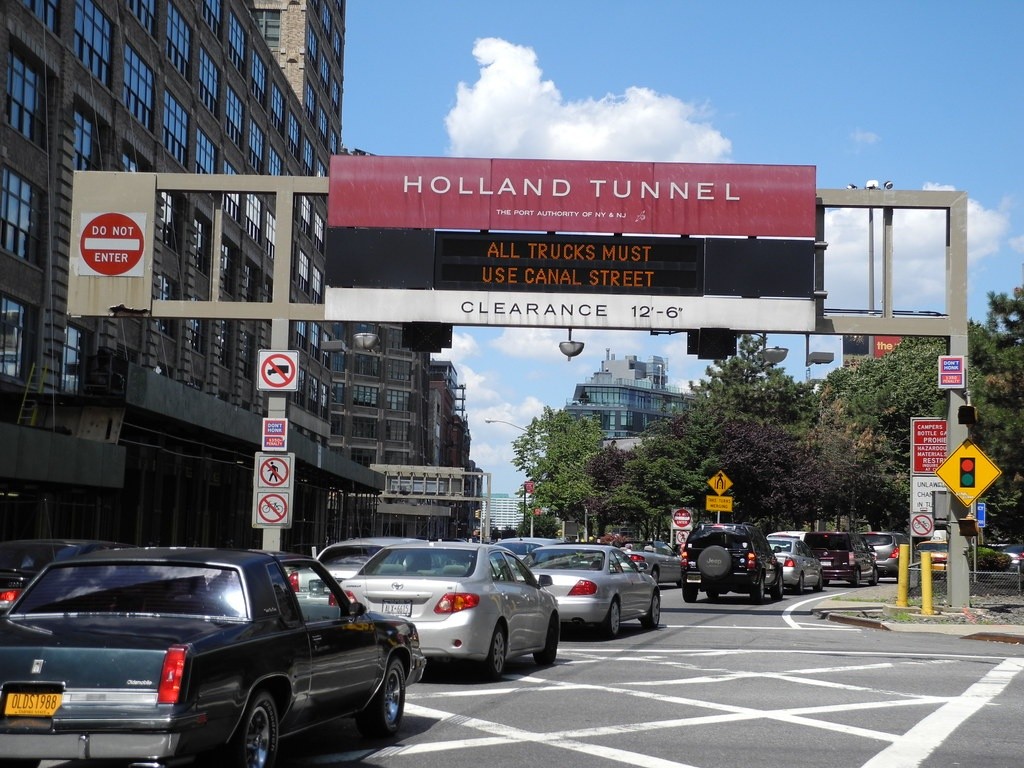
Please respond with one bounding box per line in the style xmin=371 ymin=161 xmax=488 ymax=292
xmin=959 ymin=457 xmax=975 ymax=488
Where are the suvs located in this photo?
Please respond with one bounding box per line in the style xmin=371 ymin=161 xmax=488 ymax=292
xmin=858 ymin=531 xmax=908 ymax=584
xmin=679 ymin=521 xmax=785 ymax=605
xmin=802 ymin=532 xmax=880 ymax=588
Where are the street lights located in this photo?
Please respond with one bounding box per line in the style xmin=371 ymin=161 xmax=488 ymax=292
xmin=484 ymin=416 xmax=537 ymax=537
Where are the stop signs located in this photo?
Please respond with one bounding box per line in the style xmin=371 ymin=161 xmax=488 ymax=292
xmin=75 ymin=211 xmax=147 ymax=278
xmin=670 ymin=508 xmax=694 ymax=531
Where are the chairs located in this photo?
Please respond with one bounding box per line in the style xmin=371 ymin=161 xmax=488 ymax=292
xmin=378 ymin=562 xmax=406 ymax=571
xmin=441 ymin=564 xmax=467 ymax=576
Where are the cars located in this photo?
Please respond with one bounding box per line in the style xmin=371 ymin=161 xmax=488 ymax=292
xmin=0 ymin=548 xmax=424 ymax=768
xmin=508 ymin=544 xmax=662 ymax=641
xmin=616 ymin=540 xmax=683 ymax=589
xmin=1 ymin=539 xmax=140 ymax=619
xmin=493 ymin=537 xmax=563 ymax=563
xmin=765 ymin=536 xmax=824 ymax=594
xmin=996 ymin=544 xmax=1024 ymax=573
xmin=286 ymin=535 xmax=429 ymax=626
xmin=326 ymin=541 xmax=561 ymax=682
xmin=913 ymin=540 xmax=949 ymax=579
xmin=766 ymin=530 xmax=806 ymax=542
